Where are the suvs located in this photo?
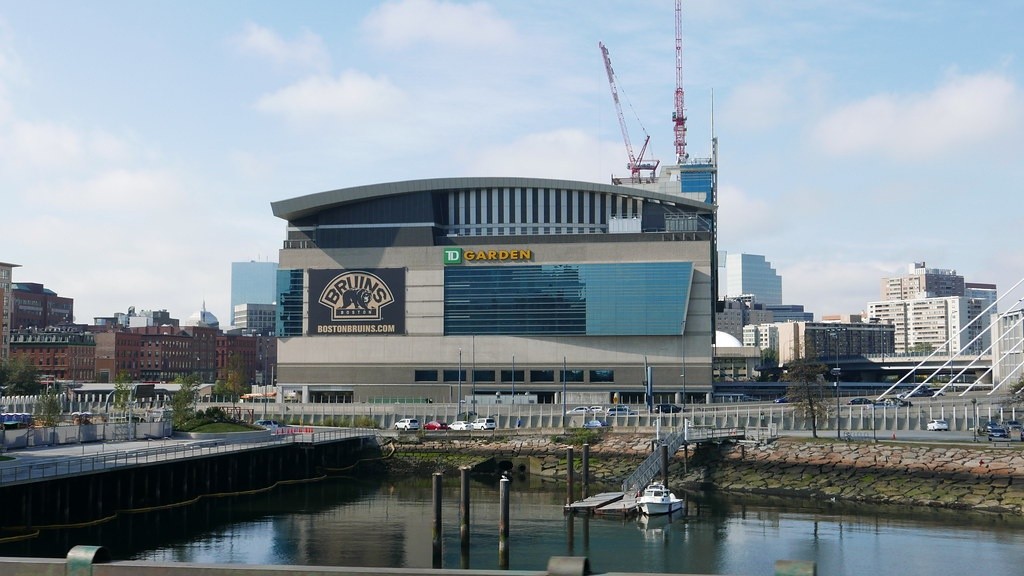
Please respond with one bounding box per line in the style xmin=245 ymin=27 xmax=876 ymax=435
xmin=394 ymin=418 xmax=419 ymax=432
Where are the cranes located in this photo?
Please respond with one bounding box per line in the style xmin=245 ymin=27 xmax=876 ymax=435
xmin=597 ymin=39 xmax=660 ymax=184
xmin=670 ymin=1 xmax=689 ymax=163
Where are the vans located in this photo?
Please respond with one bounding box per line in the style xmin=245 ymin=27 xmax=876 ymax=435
xmin=471 ymin=418 xmax=496 ymax=431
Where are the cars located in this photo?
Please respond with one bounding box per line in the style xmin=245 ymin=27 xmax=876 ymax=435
xmin=980 ymin=420 xmax=1024 ymax=443
xmin=926 ymin=419 xmax=948 ymax=431
xmin=871 ymin=387 xmax=945 ymax=408
xmin=566 ymin=404 xmax=635 ymax=416
xmin=448 ymin=420 xmax=474 ymax=431
xmin=847 ymin=397 xmax=873 ymax=404
xmin=424 ymin=420 xmax=447 ymax=431
xmin=253 ymin=419 xmax=279 ymax=429
xmin=773 ymin=395 xmax=803 ymax=403
xmin=582 ymin=420 xmax=609 ymax=428
xmin=655 ymin=404 xmax=685 ymax=413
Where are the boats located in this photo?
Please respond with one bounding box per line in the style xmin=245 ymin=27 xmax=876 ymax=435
xmin=636 ymin=478 xmax=684 ymax=515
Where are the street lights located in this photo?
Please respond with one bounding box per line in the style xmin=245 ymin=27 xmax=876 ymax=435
xmin=264 ymin=338 xmax=270 ymax=420
xmin=826 ymin=327 xmax=847 ymax=438
